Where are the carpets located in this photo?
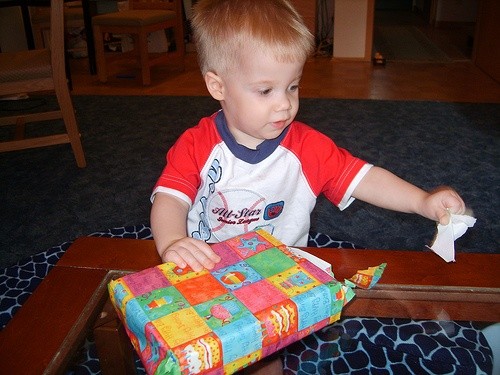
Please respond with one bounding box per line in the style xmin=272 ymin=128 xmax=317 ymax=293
xmin=376 ymin=25 xmax=471 ymax=64
xmin=0 ymin=95 xmax=500 ymax=267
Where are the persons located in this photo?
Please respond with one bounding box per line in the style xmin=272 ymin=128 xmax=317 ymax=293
xmin=148 ymin=0 xmax=464 ymax=375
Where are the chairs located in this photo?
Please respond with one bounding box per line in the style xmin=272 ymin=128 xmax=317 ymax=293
xmin=0 ymin=0 xmax=87 ymax=169
xmin=92 ymin=0 xmax=186 ymax=85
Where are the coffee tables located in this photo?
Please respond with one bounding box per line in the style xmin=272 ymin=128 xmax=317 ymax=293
xmin=0 ymin=236 xmax=500 ymax=375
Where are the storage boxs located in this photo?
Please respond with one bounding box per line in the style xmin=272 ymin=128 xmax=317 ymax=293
xmin=108 ymin=229 xmax=344 ymax=375
